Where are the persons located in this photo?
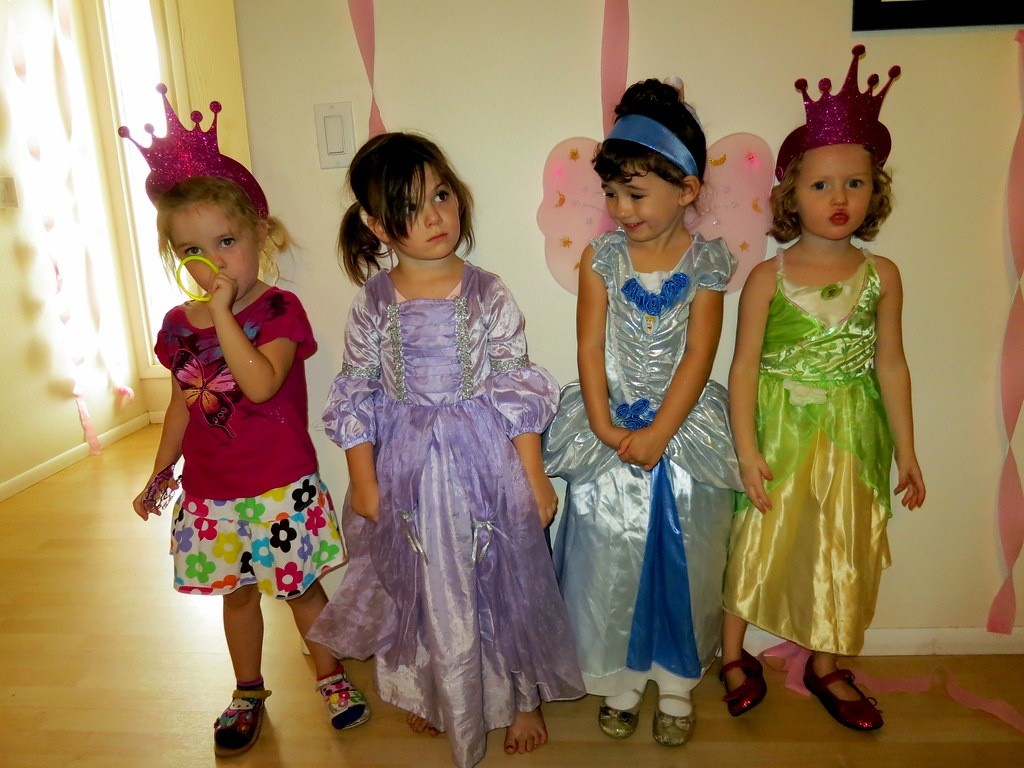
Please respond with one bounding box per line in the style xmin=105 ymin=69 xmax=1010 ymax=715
xmin=537 ymin=74 xmax=747 ymax=748
xmin=716 ymin=123 xmax=924 ymax=734
xmin=131 ymin=154 xmax=373 ymax=761
xmin=306 ymin=132 xmax=585 ymax=768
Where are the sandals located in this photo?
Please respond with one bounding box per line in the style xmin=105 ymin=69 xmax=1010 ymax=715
xmin=315 ymin=672 xmax=371 ymax=731
xmin=214 ymin=688 xmax=272 ymax=758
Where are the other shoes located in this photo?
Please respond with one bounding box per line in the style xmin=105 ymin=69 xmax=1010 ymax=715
xmin=803 ymin=655 xmax=883 ymax=730
xmin=652 ymin=686 xmax=696 ymax=746
xmin=598 ymin=685 xmax=647 ymax=739
xmin=719 ymin=648 xmax=767 ymax=717
